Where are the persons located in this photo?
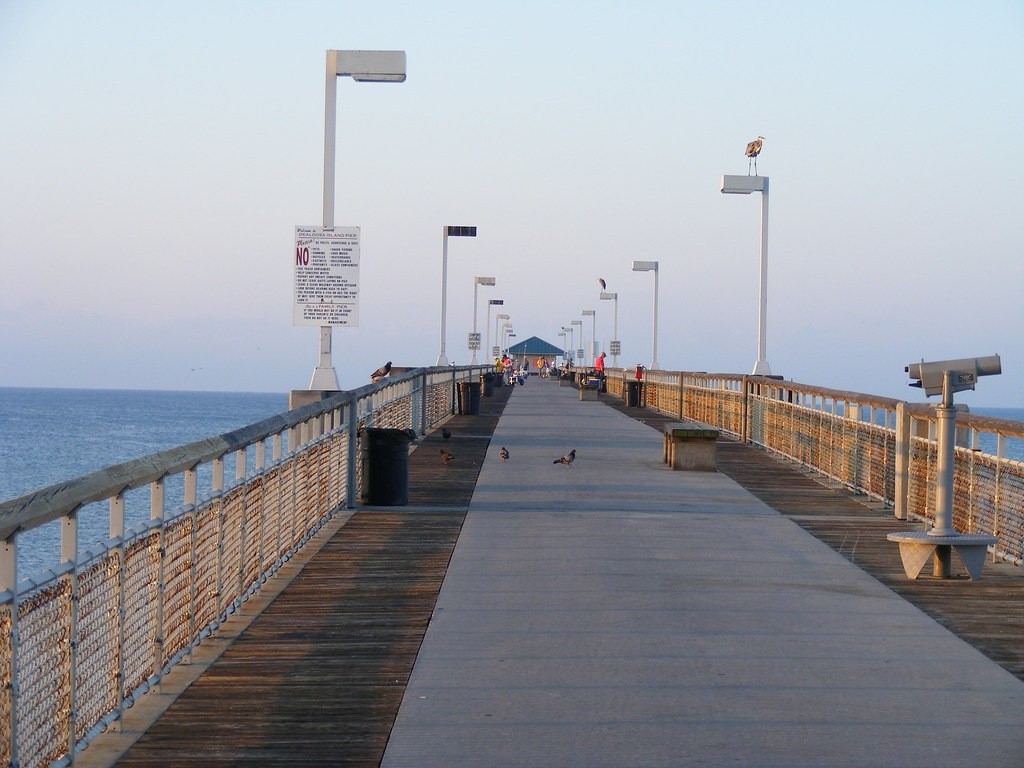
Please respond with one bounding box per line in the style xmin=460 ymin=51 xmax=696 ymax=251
xmin=558 ymin=358 xmax=574 ymax=376
xmin=595 ymin=352 xmax=606 ymax=376
xmin=494 ymin=354 xmax=529 ymax=385
xmin=536 ymin=357 xmax=555 ymax=378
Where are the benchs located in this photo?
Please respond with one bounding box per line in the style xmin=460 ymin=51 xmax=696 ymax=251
xmin=548 ymin=372 xmax=553 ymax=379
xmin=579 ymin=384 xmax=598 ymax=401
xmin=662 ymin=421 xmax=720 ymax=473
xmin=559 ymin=376 xmax=571 ymax=387
xmin=549 ymin=373 xmax=559 ymax=381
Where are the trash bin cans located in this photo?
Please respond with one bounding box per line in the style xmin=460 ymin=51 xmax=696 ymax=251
xmin=570 ymin=372 xmax=576 ymax=382
xmin=480 ymin=375 xmax=493 ymax=397
xmin=625 ymin=381 xmax=643 ymax=407
xmin=578 ymin=373 xmax=585 ymax=386
xmin=493 ymin=372 xmax=504 ymax=387
xmin=457 ymin=382 xmax=482 ymax=415
xmin=359 ymin=427 xmax=413 ymax=506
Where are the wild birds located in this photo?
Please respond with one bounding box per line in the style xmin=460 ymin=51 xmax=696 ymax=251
xmin=439 ymin=448 xmax=455 ymax=464
xmin=371 ymin=361 xmax=392 ymax=380
xmin=744 ymin=134 xmax=767 ymax=177
xmin=499 ymin=447 xmax=509 ymax=461
xmin=358 ymin=420 xmax=366 ymax=432
xmin=451 ymin=360 xmax=455 ymax=367
xmin=441 ymin=427 xmax=452 ymax=441
xmin=598 ymin=278 xmax=607 ymax=294
xmin=553 ymin=448 xmax=577 ymax=465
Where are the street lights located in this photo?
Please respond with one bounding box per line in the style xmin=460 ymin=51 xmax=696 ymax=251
xmin=571 ymin=320 xmax=584 ymax=367
xmin=632 ymin=260 xmax=659 ymax=371
xmin=436 ymin=225 xmax=477 ymax=363
xmin=557 ymin=327 xmax=573 ymax=359
xmin=307 ymin=50 xmax=408 ymax=388
xmin=470 ymin=276 xmax=496 ymax=365
xmin=493 ymin=313 xmax=516 ymax=365
xmin=719 ymin=173 xmax=771 ymax=375
xmin=483 ymin=299 xmax=504 ymax=364
xmin=599 ymin=292 xmax=620 ymax=367
xmin=581 ymin=309 xmax=597 ymax=367
